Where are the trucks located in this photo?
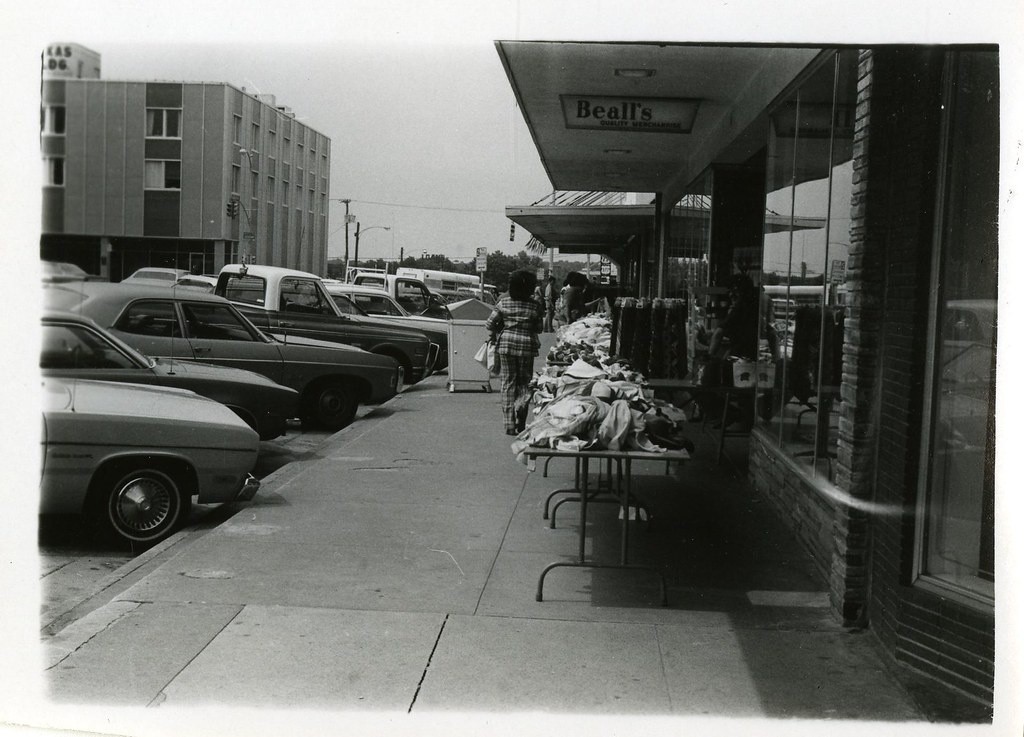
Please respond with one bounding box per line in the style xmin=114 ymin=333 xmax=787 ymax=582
xmin=396 ymin=267 xmax=497 ymax=300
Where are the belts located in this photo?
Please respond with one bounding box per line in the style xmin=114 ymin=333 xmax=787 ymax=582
xmin=609 ymin=297 xmax=687 ymax=380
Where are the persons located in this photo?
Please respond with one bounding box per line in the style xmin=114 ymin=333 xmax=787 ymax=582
xmin=707 ymin=274 xmax=760 ymax=430
xmin=484 ymin=270 xmax=544 ymax=436
xmin=533 ymin=272 xmax=604 ymax=334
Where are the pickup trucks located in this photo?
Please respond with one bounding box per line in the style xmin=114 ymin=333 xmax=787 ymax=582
xmin=213 ymin=264 xmax=439 ymax=387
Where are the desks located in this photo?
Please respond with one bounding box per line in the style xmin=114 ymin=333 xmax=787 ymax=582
xmin=519 ymin=442 xmax=691 ymax=609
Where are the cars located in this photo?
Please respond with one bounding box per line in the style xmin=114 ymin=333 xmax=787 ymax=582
xmin=177 ymin=274 xmax=218 ymax=294
xmin=318 ymin=274 xmax=451 ymax=373
xmin=46 ymin=283 xmax=404 ymax=434
xmin=119 ymin=267 xmax=191 ymax=287
xmin=942 ymin=301 xmax=995 ymax=388
xmin=453 ymin=288 xmax=497 ymax=306
xmin=41 ymin=310 xmax=260 ymax=557
xmin=42 ymin=259 xmax=88 ymax=283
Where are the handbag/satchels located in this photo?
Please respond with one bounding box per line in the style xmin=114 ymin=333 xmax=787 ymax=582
xmin=487 ymin=326 xmax=506 ymax=375
xmin=473 ymin=338 xmax=493 ymax=369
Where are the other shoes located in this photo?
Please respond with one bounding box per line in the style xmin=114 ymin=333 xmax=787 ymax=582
xmin=518 ymin=425 xmax=525 ymax=432
xmin=506 ymin=428 xmax=515 ymax=435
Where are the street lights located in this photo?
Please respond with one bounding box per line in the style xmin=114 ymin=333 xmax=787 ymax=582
xmin=354 ymin=221 xmax=391 ymax=267
xmin=340 ymin=198 xmax=351 ymax=266
